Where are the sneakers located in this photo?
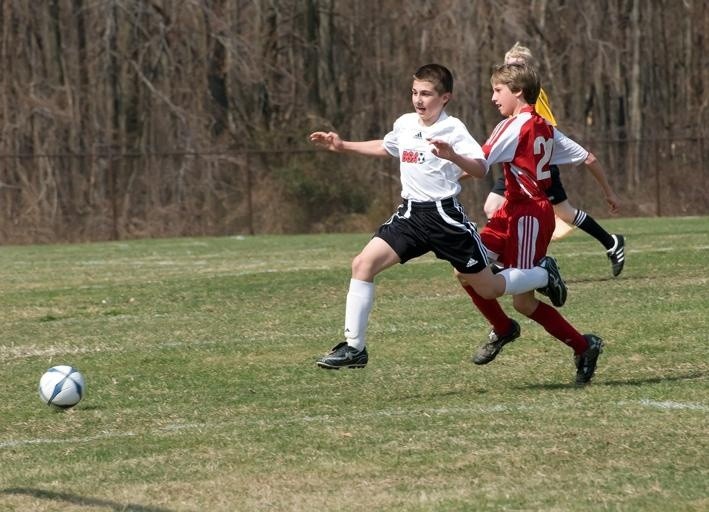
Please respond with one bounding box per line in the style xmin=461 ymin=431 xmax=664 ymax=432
xmin=574 ymin=334 xmax=605 ymax=384
xmin=607 ymin=234 xmax=626 ymax=276
xmin=473 ymin=318 xmax=521 ymax=364
xmin=536 ymin=256 xmax=567 ymax=307
xmin=316 ymin=342 xmax=369 ymax=370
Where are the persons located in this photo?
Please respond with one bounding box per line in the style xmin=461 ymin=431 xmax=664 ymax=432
xmin=482 ymin=40 xmax=626 ymax=276
xmin=309 ymin=63 xmax=522 ymax=370
xmin=453 ymin=63 xmax=604 ymax=388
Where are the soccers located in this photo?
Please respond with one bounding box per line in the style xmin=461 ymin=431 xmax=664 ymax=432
xmin=39 ymin=366 xmax=84 ymax=410
